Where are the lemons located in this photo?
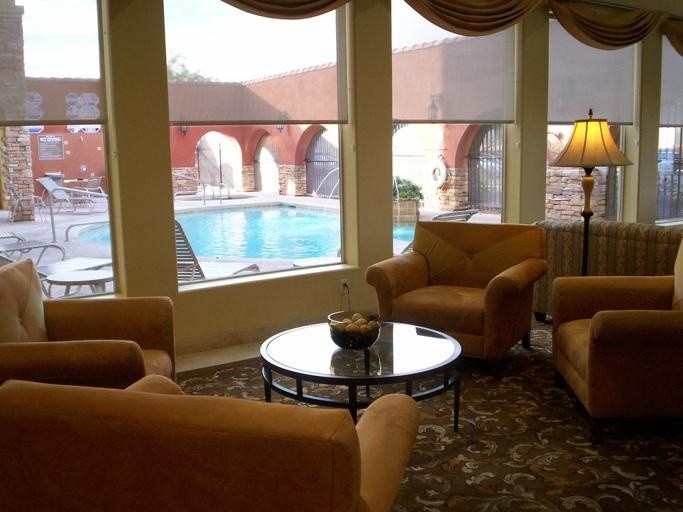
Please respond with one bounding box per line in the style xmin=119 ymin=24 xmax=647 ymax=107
xmin=333 ymin=312 xmax=379 ymax=334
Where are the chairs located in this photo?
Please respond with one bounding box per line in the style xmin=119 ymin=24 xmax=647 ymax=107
xmin=0 ymin=255 xmax=113 ymax=298
xmin=0 ymin=231 xmax=65 ymax=267
xmin=0 ymin=373 xmax=421 ymax=512
xmin=0 ymin=258 xmax=175 ymax=390
xmin=552 ymin=237 xmax=683 ymax=437
xmin=336 ymin=208 xmax=479 ymax=258
xmin=37 ymin=174 xmax=108 ymax=215
xmin=7 ymin=187 xmax=48 ymax=225
xmin=365 ymin=220 xmax=548 ymax=380
xmin=174 ymin=218 xmax=261 ymax=283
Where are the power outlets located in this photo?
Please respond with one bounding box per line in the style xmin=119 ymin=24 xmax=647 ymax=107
xmin=341 ymin=279 xmax=351 ymax=294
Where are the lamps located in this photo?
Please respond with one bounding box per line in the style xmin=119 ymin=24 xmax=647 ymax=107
xmin=277 ymin=112 xmax=286 ymax=132
xmin=427 ymin=94 xmax=443 ymax=121
xmin=548 ymin=108 xmax=633 ymax=276
xmin=181 ymin=125 xmax=188 ymax=134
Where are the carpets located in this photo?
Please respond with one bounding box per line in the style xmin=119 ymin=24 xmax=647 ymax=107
xmin=175 ymin=312 xmax=683 ymax=512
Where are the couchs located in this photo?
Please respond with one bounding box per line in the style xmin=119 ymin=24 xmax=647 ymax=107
xmin=533 ymin=220 xmax=683 ymax=321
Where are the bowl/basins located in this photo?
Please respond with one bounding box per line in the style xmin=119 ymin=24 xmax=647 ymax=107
xmin=327 ymin=310 xmax=382 ymax=350
